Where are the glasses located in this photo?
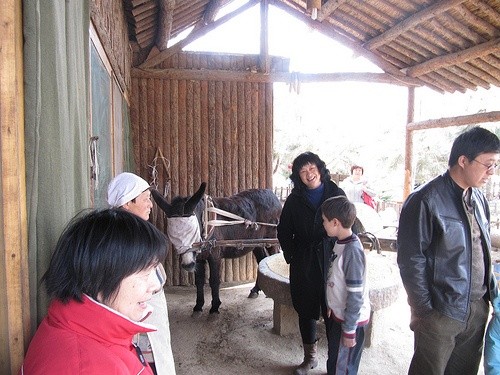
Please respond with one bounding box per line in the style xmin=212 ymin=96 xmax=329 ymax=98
xmin=472 ymin=158 xmax=500 ymax=170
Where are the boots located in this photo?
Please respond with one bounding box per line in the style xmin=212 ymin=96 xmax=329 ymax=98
xmin=295 ymin=339 xmax=319 ymax=375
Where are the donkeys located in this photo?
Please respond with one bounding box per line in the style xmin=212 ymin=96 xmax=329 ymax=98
xmin=150 ymin=181 xmax=285 ymax=321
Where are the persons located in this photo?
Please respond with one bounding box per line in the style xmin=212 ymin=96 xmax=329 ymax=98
xmin=20 ymin=206 xmax=171 ymax=375
xmin=276 ymin=151 xmax=357 ymax=375
xmin=322 ymin=195 xmax=370 ymax=375
xmin=396 ymin=127 xmax=500 ymax=375
xmin=106 ymin=172 xmax=177 ymax=375
xmin=484 ymin=294 xmax=500 ymax=375
xmin=340 ymin=164 xmax=367 ymax=203
